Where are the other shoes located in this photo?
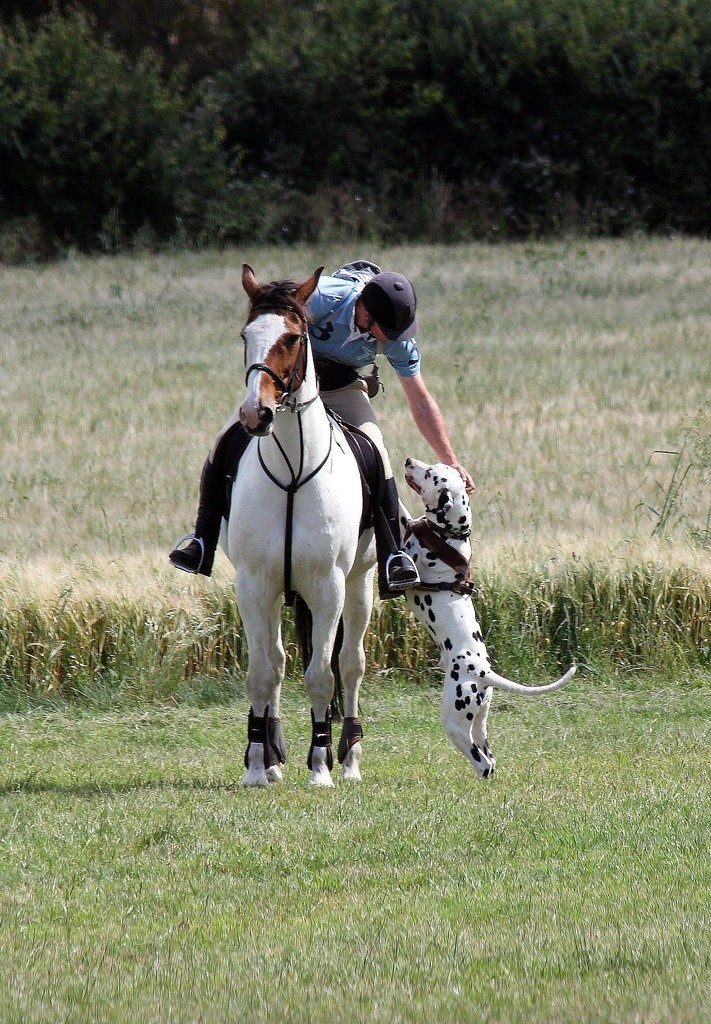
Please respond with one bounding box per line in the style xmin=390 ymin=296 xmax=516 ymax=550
xmin=379 ymin=565 xmax=417 ymax=600
xmin=169 ymin=540 xmax=214 ymax=577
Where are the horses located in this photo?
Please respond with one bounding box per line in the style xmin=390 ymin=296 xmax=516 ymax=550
xmin=206 ymin=263 xmax=413 ymax=789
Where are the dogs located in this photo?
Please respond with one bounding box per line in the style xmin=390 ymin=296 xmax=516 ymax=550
xmin=402 ymin=458 xmax=577 ymax=779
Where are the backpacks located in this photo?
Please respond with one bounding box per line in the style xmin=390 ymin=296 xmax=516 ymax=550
xmin=315 ymin=258 xmax=384 ymax=356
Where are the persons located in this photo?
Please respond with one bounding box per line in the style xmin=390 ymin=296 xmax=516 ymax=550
xmin=169 ymin=272 xmax=476 ymax=577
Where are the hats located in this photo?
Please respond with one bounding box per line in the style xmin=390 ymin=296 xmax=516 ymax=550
xmin=362 ymin=272 xmax=418 ymax=342
